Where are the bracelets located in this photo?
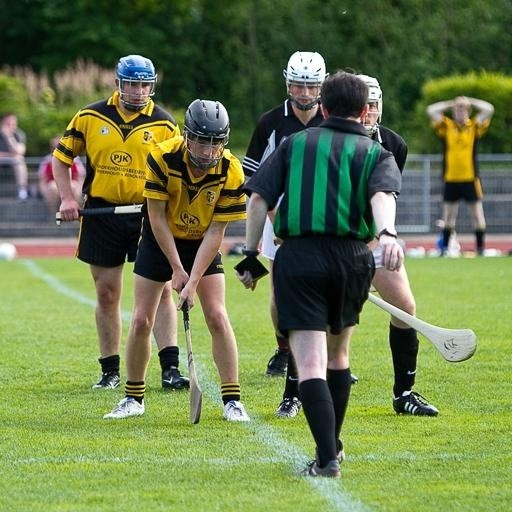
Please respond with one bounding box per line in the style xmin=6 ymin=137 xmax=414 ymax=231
xmin=240 ymin=243 xmax=263 ymax=257
xmin=377 ymin=225 xmax=400 ymax=239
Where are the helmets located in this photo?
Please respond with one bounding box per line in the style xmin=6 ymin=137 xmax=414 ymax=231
xmin=182 ymin=99 xmax=230 ymax=170
xmin=114 ymin=55 xmax=159 ymax=113
xmin=283 ymin=51 xmax=330 ymax=111
xmin=355 ymin=75 xmax=383 ymax=134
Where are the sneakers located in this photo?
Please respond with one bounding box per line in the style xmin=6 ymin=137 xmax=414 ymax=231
xmin=392 ymin=387 xmax=439 ymax=415
xmin=93 ymin=363 xmax=250 ymax=423
xmin=266 ymin=351 xmax=358 ymax=419
xmin=301 ymin=438 xmax=344 ymax=478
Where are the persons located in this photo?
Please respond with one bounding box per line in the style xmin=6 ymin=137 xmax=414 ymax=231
xmin=425 ymin=95 xmax=494 ymax=257
xmin=0 ymin=112 xmax=30 ymax=203
xmin=52 ymin=52 xmax=190 ymax=392
xmin=273 ymin=73 xmax=440 ymax=417
xmin=243 ymin=51 xmax=359 ymax=383
xmin=103 ymin=98 xmax=249 ymax=423
xmin=237 ymin=69 xmax=405 ymax=478
xmin=38 ymin=136 xmax=85 ymax=214
